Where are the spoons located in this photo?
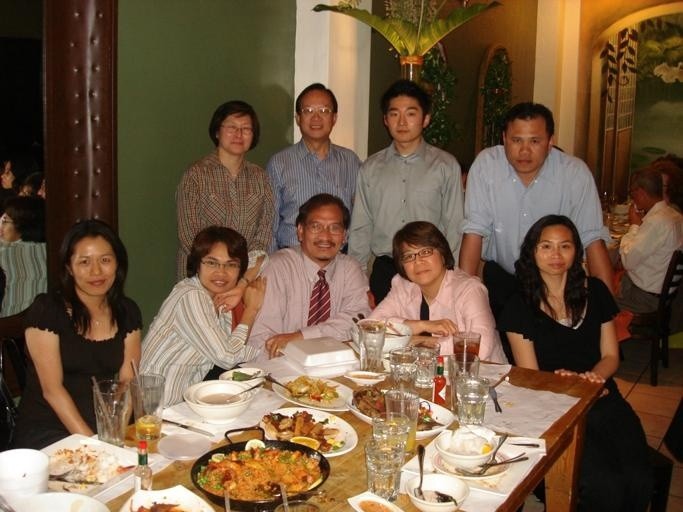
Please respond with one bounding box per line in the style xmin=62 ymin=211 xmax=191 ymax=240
xmin=414 ymin=445 xmax=425 ymax=500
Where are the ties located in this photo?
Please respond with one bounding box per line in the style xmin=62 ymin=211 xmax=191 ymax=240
xmin=307 ymin=270 xmax=330 ymax=326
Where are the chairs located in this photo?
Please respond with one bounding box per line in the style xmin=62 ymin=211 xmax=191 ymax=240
xmin=1 ymin=334 xmax=27 ymax=451
xmin=620 ymin=249 xmax=683 ymax=386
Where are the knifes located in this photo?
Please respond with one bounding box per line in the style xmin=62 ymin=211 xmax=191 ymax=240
xmin=49 ymin=474 xmax=104 ymax=485
xmin=489 ymin=387 xmax=502 ymax=415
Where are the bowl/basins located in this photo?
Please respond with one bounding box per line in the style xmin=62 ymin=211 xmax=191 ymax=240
xmin=195 ymin=385 xmax=247 ymax=404
xmin=220 ymin=367 xmax=263 ymax=383
xmin=0 ymin=448 xmax=49 ymax=500
xmin=406 ymin=474 xmax=470 ymax=512
xmin=434 ymin=424 xmax=497 ymax=469
xmin=183 ymin=380 xmax=257 ymax=423
xmin=351 ymin=320 xmax=412 ymax=355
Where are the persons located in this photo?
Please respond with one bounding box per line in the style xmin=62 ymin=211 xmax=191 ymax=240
xmin=1 ymin=79 xmax=683 ymax=512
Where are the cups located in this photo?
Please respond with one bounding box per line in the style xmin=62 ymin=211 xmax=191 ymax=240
xmin=365 ymin=437 xmax=404 ymax=502
xmin=274 ymin=500 xmax=320 ymax=512
xmin=413 ymin=342 xmax=440 ymax=388
xmin=130 ymin=374 xmax=166 ymax=441
xmin=385 ymin=391 xmax=419 ymax=455
xmin=389 ymin=347 xmax=418 ymax=390
xmin=452 ymin=331 xmax=481 ymax=373
xmin=373 ymin=413 xmax=409 ymax=468
xmin=357 ymin=320 xmax=384 ymax=371
xmin=449 ymin=353 xmax=480 ymax=410
xmin=93 ymin=380 xmax=129 ymax=447
xmin=456 ymin=376 xmax=489 ymax=425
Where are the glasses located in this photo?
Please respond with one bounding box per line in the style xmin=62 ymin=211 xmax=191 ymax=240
xmin=302 ymin=106 xmax=331 ymax=114
xmin=1 ymin=217 xmax=13 ymax=223
xmin=221 ymin=125 xmax=252 ymax=134
xmin=400 ymin=248 xmax=433 ymax=263
xmin=303 ymin=222 xmax=345 ymax=235
xmin=201 ymin=260 xmax=239 ymax=269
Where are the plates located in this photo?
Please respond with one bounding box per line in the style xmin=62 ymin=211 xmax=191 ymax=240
xmin=118 ymin=485 xmax=215 ymax=512
xmin=432 ymin=452 xmax=511 ymax=479
xmin=347 ymin=491 xmax=404 ymax=512
xmin=346 ymin=390 xmax=454 ymax=440
xmin=40 ymin=434 xmax=154 ymax=498
xmin=157 ymin=433 xmax=210 ymax=460
xmin=272 ymin=376 xmax=352 ymax=411
xmin=260 ymin=407 xmax=358 ymax=458
xmin=19 ymin=493 xmax=111 ymax=512
xmin=344 ymin=371 xmax=385 ymax=386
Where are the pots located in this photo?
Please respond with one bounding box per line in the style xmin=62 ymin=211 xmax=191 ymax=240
xmin=191 ymin=426 xmax=330 ymax=512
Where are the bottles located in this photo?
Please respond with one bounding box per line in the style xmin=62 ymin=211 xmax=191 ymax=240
xmin=432 ymin=356 xmax=446 ymax=405
xmin=135 ymin=440 xmax=152 ymax=492
